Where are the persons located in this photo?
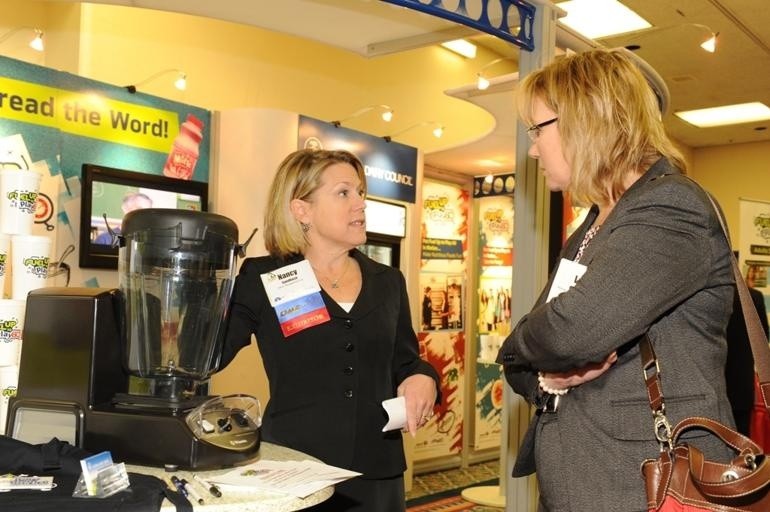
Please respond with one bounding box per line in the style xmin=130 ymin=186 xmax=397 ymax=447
xmin=479 ymin=287 xmax=510 ymax=330
xmin=422 ymin=286 xmax=439 ymax=326
xmin=95 ymin=194 xmax=152 ymax=245
xmin=726 ymin=248 xmax=770 ymax=437
xmin=495 ymin=45 xmax=739 ymax=512
xmin=438 ymin=289 xmax=455 ymax=330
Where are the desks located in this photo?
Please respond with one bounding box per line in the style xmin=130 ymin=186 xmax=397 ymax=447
xmin=114 ymin=438 xmax=335 ymax=512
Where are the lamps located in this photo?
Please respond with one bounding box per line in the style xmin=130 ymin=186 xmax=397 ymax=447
xmin=0 ymin=23 xmax=45 ymax=53
xmin=477 ymin=53 xmax=519 ymax=90
xmin=383 ymin=119 xmax=448 ymax=143
xmin=121 ymin=68 xmax=189 ymax=94
xmin=687 ymin=20 xmax=720 ymax=54
xmin=328 ymin=103 xmax=395 ymax=129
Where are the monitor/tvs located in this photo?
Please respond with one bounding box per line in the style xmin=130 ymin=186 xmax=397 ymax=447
xmin=80 ymin=164 xmax=208 ymax=267
xmin=355 ymin=239 xmax=400 ymax=271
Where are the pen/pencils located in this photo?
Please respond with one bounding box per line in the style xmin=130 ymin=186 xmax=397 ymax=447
xmin=181 ymin=479 xmax=203 ymax=505
xmin=193 ymin=474 xmax=221 ymax=497
xmin=172 ymin=476 xmax=188 ymax=498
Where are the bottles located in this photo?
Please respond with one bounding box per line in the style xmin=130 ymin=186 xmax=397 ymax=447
xmin=162 ymin=113 xmax=206 ymax=181
xmin=479 ymin=285 xmax=511 ymax=331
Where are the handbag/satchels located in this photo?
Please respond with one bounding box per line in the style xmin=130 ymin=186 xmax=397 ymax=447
xmin=639 ymin=418 xmax=770 ymax=512
xmin=0 ymin=435 xmax=192 ymax=511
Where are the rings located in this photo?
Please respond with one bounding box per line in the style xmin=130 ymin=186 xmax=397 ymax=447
xmin=422 ymin=413 xmax=432 ymax=421
xmin=183 ymin=150 xmax=443 ymax=511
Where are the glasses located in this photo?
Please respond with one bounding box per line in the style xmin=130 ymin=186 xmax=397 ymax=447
xmin=526 ymin=118 xmax=558 ymax=141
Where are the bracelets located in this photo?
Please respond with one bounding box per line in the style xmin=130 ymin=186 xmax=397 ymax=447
xmin=535 ymin=367 xmax=569 ymax=399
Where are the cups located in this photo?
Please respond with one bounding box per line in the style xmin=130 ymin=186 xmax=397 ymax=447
xmin=10 ymin=233 xmax=51 ymax=303
xmin=3 ymin=299 xmax=25 ymax=366
xmin=480 ymin=334 xmax=510 ymax=363
xmin=2 ymin=170 xmax=40 ymax=252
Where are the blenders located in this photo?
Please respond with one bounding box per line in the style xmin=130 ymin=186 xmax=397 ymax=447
xmin=3 ymin=208 xmax=262 ymax=469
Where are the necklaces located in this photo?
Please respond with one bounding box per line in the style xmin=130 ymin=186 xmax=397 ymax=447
xmin=308 ymin=258 xmax=350 ymax=289
